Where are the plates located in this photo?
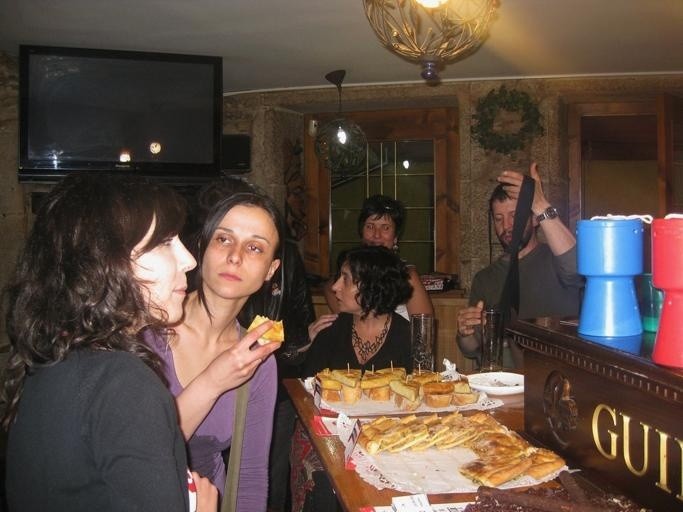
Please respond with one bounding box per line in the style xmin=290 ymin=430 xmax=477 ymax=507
xmin=468 ymin=372 xmax=524 ymax=395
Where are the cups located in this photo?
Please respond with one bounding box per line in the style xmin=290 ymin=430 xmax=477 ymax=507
xmin=411 ymin=314 xmax=435 ymax=373
xmin=480 ymin=311 xmax=504 ymax=371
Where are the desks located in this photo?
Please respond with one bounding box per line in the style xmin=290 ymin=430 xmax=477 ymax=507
xmin=502 ymin=315 xmax=683 ymax=511
xmin=310 ymin=286 xmax=480 ymax=373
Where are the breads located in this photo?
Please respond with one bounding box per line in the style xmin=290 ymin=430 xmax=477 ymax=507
xmin=247 ymin=316 xmax=285 ymax=345
xmin=313 ymin=363 xmax=480 ymax=413
xmin=355 ymin=408 xmax=565 ymax=487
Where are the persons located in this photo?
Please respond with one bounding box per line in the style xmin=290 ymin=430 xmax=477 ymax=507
xmin=197 ymin=176 xmax=315 ymax=348
xmin=323 ymin=193 xmax=434 ymax=328
xmin=0 ymin=164 xmax=218 ymax=511
xmin=143 ymin=186 xmax=286 ymax=511
xmin=457 ymin=162 xmax=584 ymax=368
xmin=274 ymin=312 xmax=338 ymax=368
xmin=287 ymin=239 xmax=429 ymax=511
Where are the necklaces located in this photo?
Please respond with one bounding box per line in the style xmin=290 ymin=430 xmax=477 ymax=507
xmin=349 ymin=316 xmax=392 ymax=362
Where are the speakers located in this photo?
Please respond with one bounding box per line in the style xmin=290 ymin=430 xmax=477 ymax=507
xmin=224 ymin=133 xmax=250 ymax=173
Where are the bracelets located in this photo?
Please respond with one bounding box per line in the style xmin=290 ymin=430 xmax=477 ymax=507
xmin=535 ymin=205 xmax=559 ymax=221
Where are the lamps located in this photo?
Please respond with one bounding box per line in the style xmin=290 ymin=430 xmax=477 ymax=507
xmin=314 ymin=69 xmax=368 ymax=175
xmin=361 ymin=0 xmax=501 ymax=84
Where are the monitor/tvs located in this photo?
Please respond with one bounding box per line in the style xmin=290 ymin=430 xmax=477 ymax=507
xmin=17 ymin=44 xmax=224 ymax=181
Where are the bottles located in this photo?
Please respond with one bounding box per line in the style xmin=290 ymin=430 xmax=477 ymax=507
xmin=637 ymin=273 xmax=664 ymax=332
